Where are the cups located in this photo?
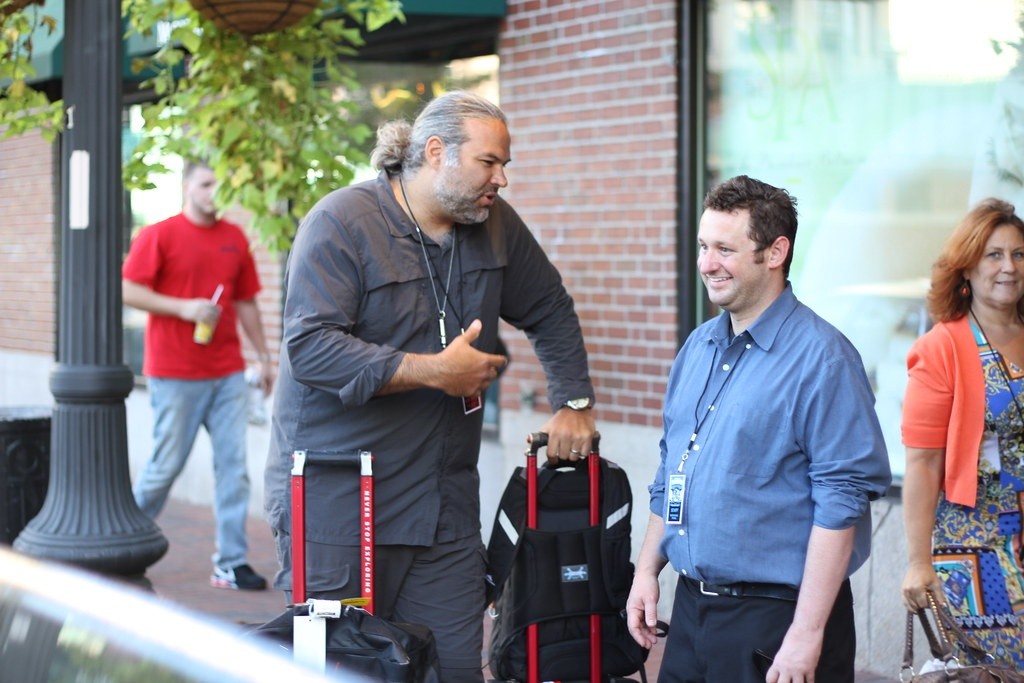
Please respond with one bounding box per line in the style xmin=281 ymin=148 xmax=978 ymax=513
xmin=193 ymin=305 xmax=222 ymax=346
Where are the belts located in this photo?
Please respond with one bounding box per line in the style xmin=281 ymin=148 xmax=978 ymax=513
xmin=695 ymin=579 xmax=845 ymax=603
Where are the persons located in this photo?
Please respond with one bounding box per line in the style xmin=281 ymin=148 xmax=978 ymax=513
xmin=625 ymin=175 xmax=894 ymax=683
xmin=258 ymin=89 xmax=599 ymax=683
xmin=120 ymin=158 xmax=276 ymax=593
xmin=897 ymin=199 xmax=1024 ymax=683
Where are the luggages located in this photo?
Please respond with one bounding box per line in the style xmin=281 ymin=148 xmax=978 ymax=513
xmin=483 ymin=433 xmax=650 ymax=682
xmin=241 ymin=446 xmax=444 ymax=683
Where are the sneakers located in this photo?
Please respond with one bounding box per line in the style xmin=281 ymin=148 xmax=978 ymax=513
xmin=211 ymin=565 xmax=266 ymax=590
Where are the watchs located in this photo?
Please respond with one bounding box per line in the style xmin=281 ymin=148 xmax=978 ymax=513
xmin=560 ymin=395 xmax=595 ymax=413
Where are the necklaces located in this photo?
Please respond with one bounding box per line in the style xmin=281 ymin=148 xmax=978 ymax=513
xmin=411 ymin=220 xmax=459 ymax=349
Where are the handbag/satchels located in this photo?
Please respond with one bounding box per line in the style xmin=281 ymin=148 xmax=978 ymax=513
xmin=898 ymin=590 xmax=1024 ymax=682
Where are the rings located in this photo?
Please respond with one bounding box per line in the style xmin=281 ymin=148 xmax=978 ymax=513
xmin=571 ymin=449 xmax=581 ymax=454
